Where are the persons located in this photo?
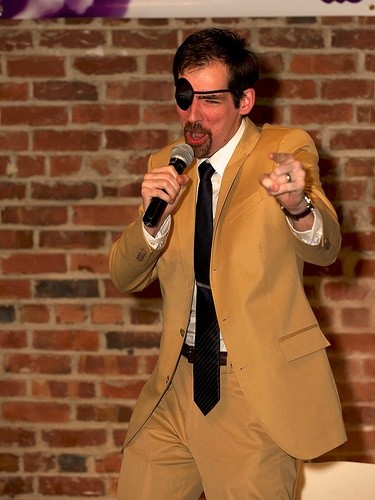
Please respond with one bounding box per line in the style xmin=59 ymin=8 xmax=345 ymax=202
xmin=108 ymin=28 xmax=348 ymax=499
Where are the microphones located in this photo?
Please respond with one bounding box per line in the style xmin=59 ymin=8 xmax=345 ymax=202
xmin=142 ymin=144 xmax=195 ymax=227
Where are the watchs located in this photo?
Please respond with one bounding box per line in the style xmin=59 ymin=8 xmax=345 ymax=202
xmin=277 ymin=191 xmax=315 ymax=220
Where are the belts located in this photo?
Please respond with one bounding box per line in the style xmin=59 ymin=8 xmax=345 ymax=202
xmin=180 ymin=342 xmax=227 ymax=366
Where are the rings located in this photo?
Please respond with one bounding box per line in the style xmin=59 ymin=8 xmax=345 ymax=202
xmin=286 ymin=172 xmax=291 ymax=181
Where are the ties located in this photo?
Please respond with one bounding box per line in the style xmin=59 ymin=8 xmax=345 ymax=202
xmin=193 ymin=160 xmax=220 ymax=416
